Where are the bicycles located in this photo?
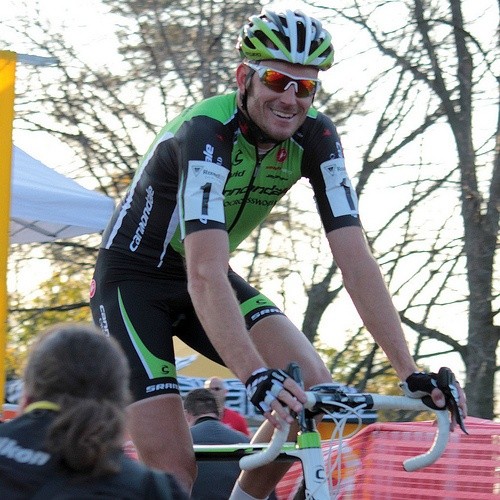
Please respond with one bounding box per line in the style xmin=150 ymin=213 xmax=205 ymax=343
xmin=121 ymin=362 xmax=470 ymax=499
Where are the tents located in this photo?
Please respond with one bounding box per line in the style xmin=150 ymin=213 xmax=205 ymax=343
xmin=10 ymin=146 xmax=115 ymax=243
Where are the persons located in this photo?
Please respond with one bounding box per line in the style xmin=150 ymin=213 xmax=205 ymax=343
xmin=204 ymin=377 xmax=249 ymax=437
xmin=0 ymin=322 xmax=188 ymax=500
xmin=88 ymin=9 xmax=468 ymax=500
xmin=184 ymin=388 xmax=250 ymax=445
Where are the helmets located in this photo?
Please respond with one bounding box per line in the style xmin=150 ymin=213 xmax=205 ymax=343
xmin=236 ymin=9 xmax=335 ymax=70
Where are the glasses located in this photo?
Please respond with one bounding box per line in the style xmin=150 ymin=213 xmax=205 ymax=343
xmin=205 ymin=387 xmax=225 ymax=392
xmin=245 ymin=63 xmax=323 ymax=98
